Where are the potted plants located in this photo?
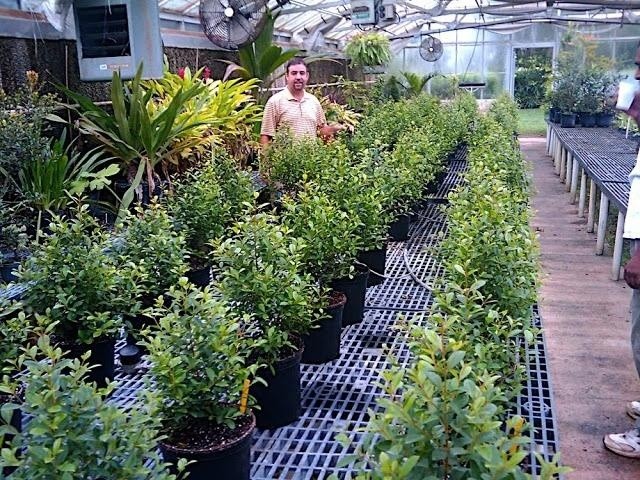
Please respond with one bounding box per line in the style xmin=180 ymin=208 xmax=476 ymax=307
xmin=543 ymin=21 xmax=621 ymax=128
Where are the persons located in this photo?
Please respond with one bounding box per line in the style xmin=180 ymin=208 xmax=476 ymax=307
xmin=603 ymin=37 xmax=640 ymax=459
xmin=256 ymin=60 xmax=354 ymax=189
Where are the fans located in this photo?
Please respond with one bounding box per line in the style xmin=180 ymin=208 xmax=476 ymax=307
xmin=198 ymin=0 xmax=271 ymax=53
xmin=420 ymin=37 xmax=443 ymax=62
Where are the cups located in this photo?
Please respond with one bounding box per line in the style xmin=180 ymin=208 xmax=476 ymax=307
xmin=616 ymin=80 xmax=638 ymax=111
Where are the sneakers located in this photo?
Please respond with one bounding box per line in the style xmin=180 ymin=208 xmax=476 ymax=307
xmin=624 ymin=398 xmax=639 ymax=421
xmin=602 ymin=424 xmax=640 ymax=459
